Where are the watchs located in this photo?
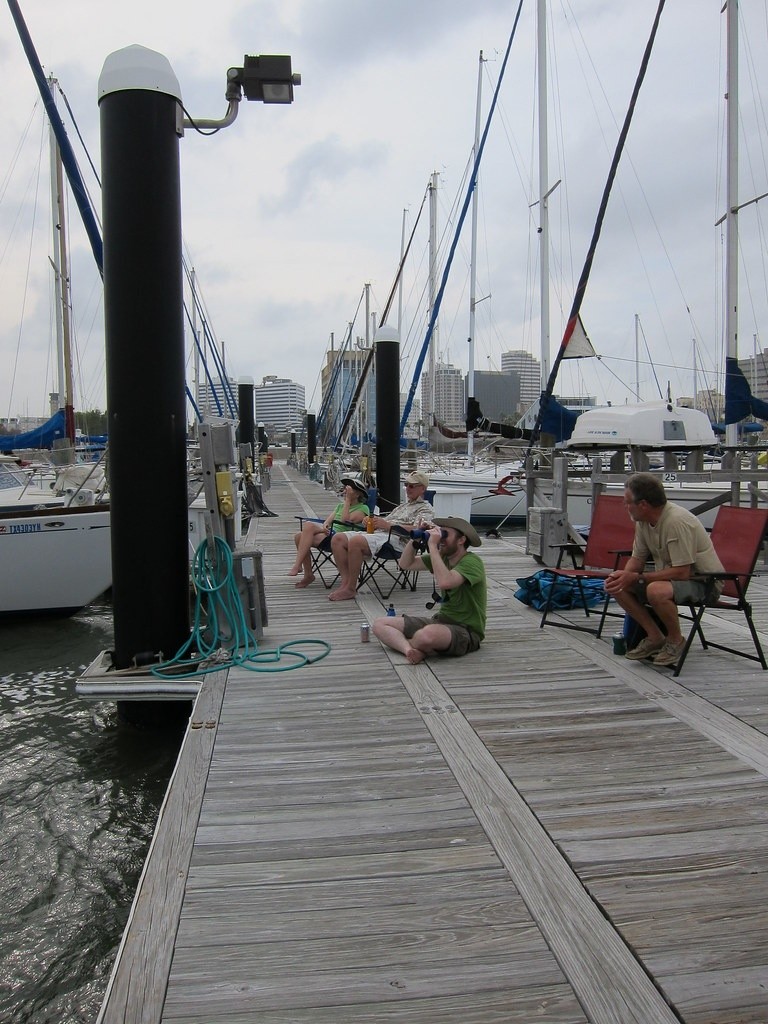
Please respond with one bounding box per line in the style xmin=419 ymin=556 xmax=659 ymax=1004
xmin=638 ymin=572 xmax=644 ymax=585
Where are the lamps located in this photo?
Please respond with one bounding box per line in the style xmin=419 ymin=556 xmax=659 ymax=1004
xmin=175 ymin=54 xmax=302 ymax=135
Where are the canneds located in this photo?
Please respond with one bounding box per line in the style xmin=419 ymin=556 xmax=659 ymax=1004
xmin=360 ymin=623 xmax=370 ymax=642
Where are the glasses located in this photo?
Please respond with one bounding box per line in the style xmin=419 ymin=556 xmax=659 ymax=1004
xmin=623 ymin=499 xmax=641 ymax=506
xmin=404 ymin=483 xmax=423 ymax=489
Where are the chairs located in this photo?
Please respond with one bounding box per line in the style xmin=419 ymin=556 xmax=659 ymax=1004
xmin=540 ymin=494 xmax=768 ymax=678
xmin=294 ymin=488 xmax=436 ymax=599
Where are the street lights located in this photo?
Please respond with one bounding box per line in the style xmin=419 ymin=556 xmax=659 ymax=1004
xmin=99 ymin=55 xmax=299 ymax=726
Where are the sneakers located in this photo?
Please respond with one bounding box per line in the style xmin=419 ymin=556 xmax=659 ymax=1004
xmin=653 ymin=637 xmax=687 ymax=666
xmin=625 ymin=637 xmax=666 ymax=660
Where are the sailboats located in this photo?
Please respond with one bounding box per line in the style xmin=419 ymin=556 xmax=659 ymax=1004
xmin=0 ymin=74 xmax=241 ymax=621
xmin=294 ymin=0 xmax=767 ymax=531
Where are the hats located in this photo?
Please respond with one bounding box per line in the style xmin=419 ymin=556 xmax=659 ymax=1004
xmin=404 ymin=471 xmax=429 ymax=488
xmin=340 ymin=478 xmax=369 ymax=501
xmin=431 ymin=517 xmax=482 ymax=547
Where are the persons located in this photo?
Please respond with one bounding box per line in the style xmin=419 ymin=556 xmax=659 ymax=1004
xmin=287 ymin=479 xmax=370 ymax=588
xmin=604 ymin=471 xmax=725 ymax=666
xmin=328 ymin=472 xmax=435 ymax=600
xmin=373 ymin=517 xmax=488 ymax=663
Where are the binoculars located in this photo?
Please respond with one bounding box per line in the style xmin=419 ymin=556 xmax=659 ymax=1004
xmin=410 ymin=528 xmax=448 ymax=542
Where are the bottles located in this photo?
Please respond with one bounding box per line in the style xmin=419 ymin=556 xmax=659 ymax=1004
xmin=329 ymin=527 xmax=336 ymax=535
xmin=367 ymin=511 xmax=374 ymax=534
xmin=387 ymin=604 xmax=396 ymax=616
